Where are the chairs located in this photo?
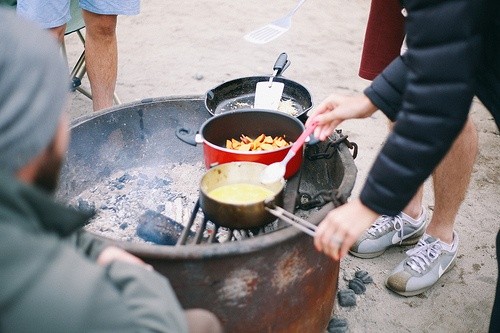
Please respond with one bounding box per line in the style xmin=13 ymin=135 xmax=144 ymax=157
xmin=63 ymin=0 xmax=121 ymax=105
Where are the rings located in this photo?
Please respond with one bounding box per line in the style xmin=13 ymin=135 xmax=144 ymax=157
xmin=332 ymin=234 xmax=342 ymax=243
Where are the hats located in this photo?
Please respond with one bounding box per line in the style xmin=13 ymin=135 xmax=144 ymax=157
xmin=0 ymin=7 xmax=68 ymax=168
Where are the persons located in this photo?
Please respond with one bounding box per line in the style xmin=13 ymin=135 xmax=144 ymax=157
xmin=306 ymin=1 xmax=500 ymax=333
xmin=16 ymin=0 xmax=141 ymax=111
xmin=0 ymin=5 xmax=225 ymax=333
xmin=347 ymin=0 xmax=476 ymax=296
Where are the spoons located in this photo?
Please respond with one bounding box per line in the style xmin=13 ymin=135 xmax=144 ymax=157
xmin=258 ymin=121 xmax=317 ymax=185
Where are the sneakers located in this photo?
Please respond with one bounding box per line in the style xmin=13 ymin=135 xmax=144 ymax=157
xmin=348 ymin=206 xmax=426 ymax=259
xmin=385 ymin=229 xmax=459 ymax=297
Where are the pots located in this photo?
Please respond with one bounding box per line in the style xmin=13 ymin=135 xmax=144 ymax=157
xmin=203 ymin=59 xmax=313 ymax=118
xmin=199 ymin=108 xmax=306 ymax=178
xmin=198 ymin=161 xmax=317 ymax=242
